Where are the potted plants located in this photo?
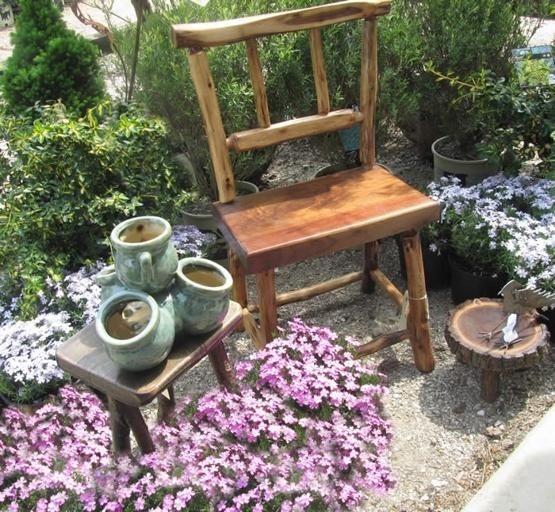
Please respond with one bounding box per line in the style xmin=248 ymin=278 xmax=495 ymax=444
xmin=421 ymin=57 xmax=555 ymax=190
xmin=419 ymin=198 xmax=514 ymax=307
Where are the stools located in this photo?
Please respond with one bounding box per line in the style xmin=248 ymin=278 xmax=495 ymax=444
xmin=56 ymin=300 xmax=245 ymax=456
xmin=445 ymin=296 xmax=552 ymax=402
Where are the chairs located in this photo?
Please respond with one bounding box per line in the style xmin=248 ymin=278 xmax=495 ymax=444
xmin=172 ymin=2 xmax=445 ymax=373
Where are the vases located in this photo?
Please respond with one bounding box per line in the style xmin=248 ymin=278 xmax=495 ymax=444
xmin=93 ymin=214 xmax=236 ymax=372
xmin=421 ymin=241 xmax=555 ymax=346
